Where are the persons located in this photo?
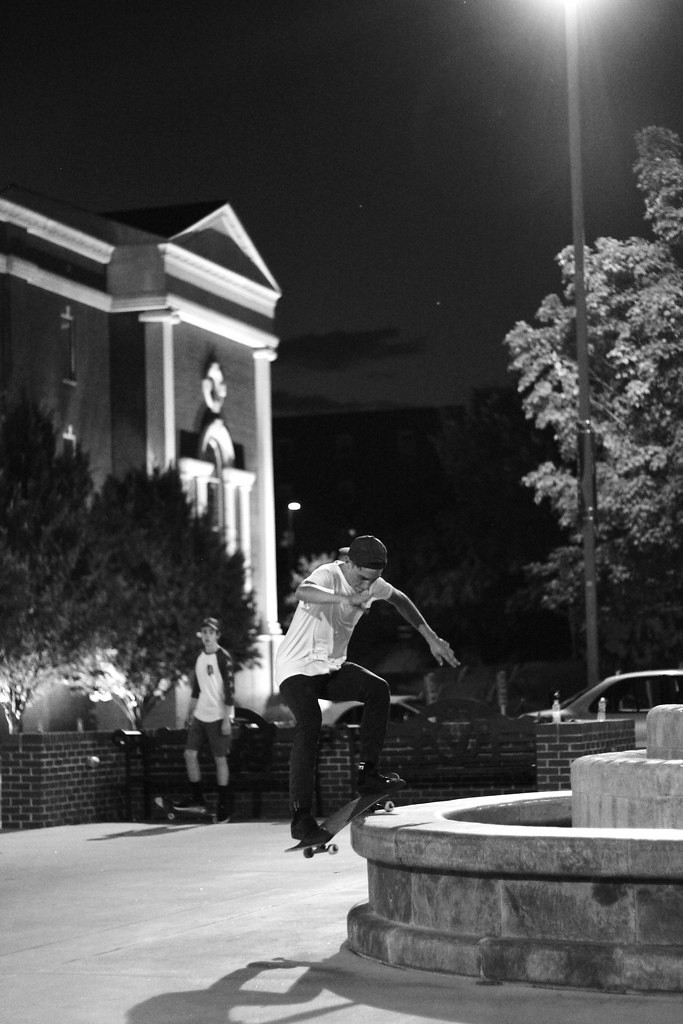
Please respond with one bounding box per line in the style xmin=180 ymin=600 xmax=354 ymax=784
xmin=172 ymin=617 xmax=235 ymax=824
xmin=273 ymin=534 xmax=460 ymax=844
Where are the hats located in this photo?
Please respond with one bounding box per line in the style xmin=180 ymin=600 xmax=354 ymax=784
xmin=339 ymin=536 xmax=387 ymax=569
xmin=201 ymin=616 xmax=219 ymax=630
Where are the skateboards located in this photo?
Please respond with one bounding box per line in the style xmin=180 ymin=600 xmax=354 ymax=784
xmin=283 ymin=772 xmax=401 ymax=859
xmin=154 ymin=796 xmax=217 ymax=824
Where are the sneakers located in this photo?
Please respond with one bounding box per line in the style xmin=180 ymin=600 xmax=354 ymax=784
xmin=174 ymin=793 xmax=207 ymax=813
xmin=291 ymin=818 xmax=334 ymax=843
xmin=357 ymin=773 xmax=405 ymax=794
xmin=215 ymin=799 xmax=235 ymax=824
